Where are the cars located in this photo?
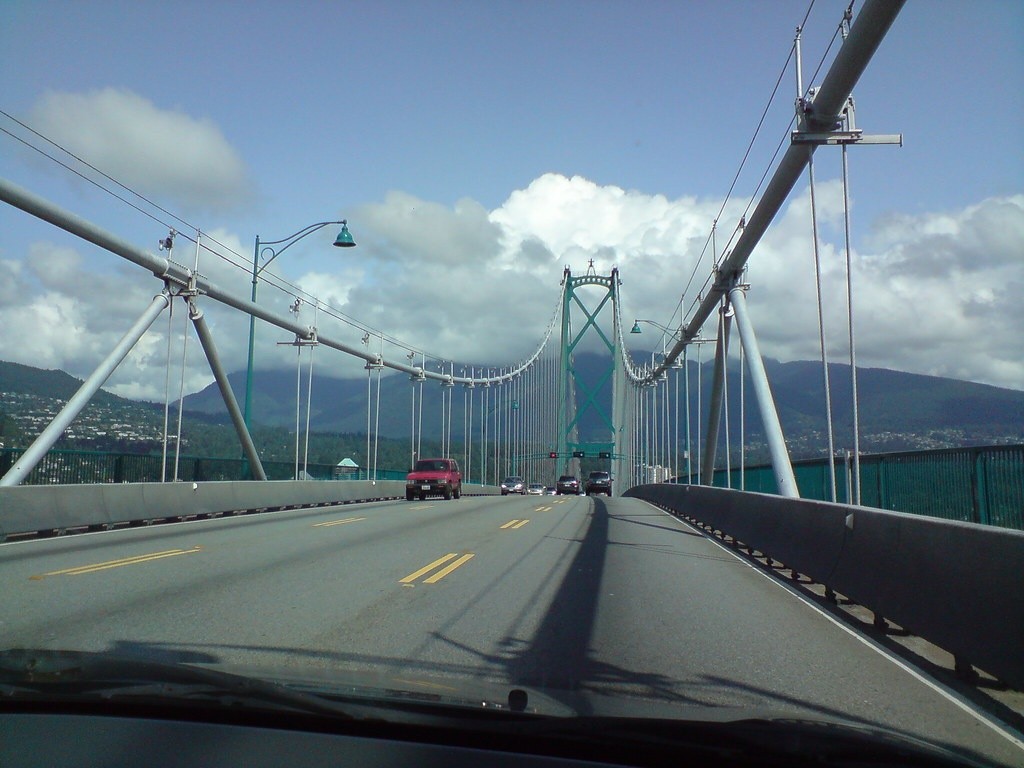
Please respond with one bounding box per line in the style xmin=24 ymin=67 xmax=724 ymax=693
xmin=527 ymin=482 xmax=557 ymax=496
xmin=501 ymin=476 xmax=527 ymax=495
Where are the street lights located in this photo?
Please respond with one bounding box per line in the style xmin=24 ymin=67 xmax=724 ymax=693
xmin=483 ymin=399 xmax=519 ymax=483
xmin=239 ymin=218 xmax=359 ymax=479
xmin=628 ymin=319 xmax=689 ymax=478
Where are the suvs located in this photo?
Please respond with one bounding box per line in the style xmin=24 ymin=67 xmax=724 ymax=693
xmin=585 ymin=470 xmax=615 ymax=498
xmin=556 ymin=475 xmax=581 ymax=495
xmin=404 ymin=458 xmax=462 ymax=500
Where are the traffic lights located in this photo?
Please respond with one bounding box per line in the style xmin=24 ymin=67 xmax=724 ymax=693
xmin=573 ymin=451 xmax=585 ymax=458
xmin=599 ymin=452 xmax=610 ymax=458
xmin=549 ymin=452 xmax=556 ymax=458
